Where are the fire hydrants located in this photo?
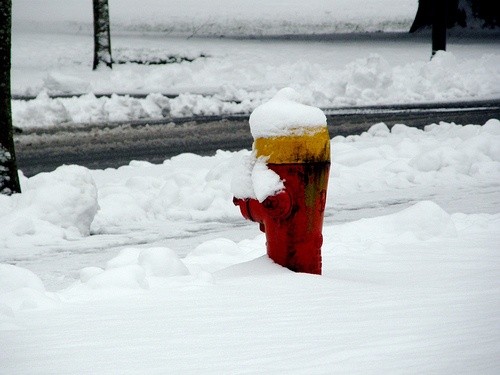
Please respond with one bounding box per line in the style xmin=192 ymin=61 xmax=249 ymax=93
xmin=233 ymin=123 xmax=330 ymax=274
